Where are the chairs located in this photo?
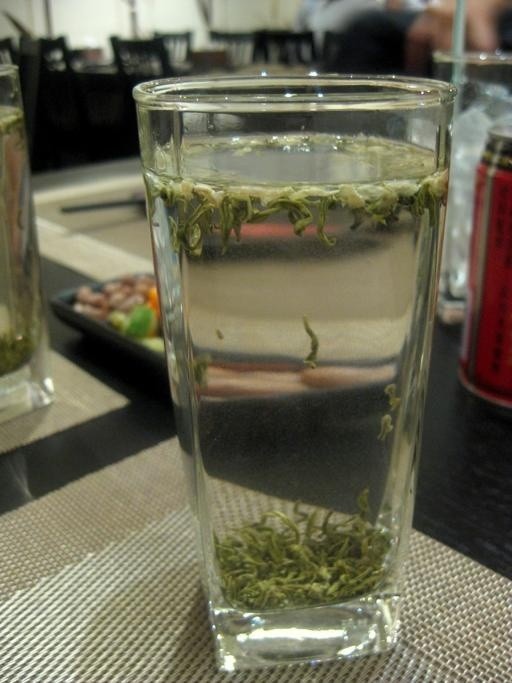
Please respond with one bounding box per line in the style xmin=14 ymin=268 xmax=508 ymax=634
xmin=3 ymin=28 xmax=373 ymax=147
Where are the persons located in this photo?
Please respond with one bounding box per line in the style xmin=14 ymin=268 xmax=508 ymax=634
xmin=292 ymin=0 xmax=426 ymax=53
xmin=341 ymin=0 xmax=510 ymax=104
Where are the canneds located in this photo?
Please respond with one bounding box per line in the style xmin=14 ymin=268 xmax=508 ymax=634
xmin=457 ymin=122 xmax=512 ymax=409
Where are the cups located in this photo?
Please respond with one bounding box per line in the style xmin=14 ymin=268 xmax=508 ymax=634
xmin=0 ymin=63 xmax=56 ymax=426
xmin=131 ymin=70 xmax=459 ymax=670
xmin=433 ymin=47 xmax=512 ymax=328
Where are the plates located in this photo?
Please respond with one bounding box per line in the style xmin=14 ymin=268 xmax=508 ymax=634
xmin=49 ymin=270 xmax=167 ymax=375
